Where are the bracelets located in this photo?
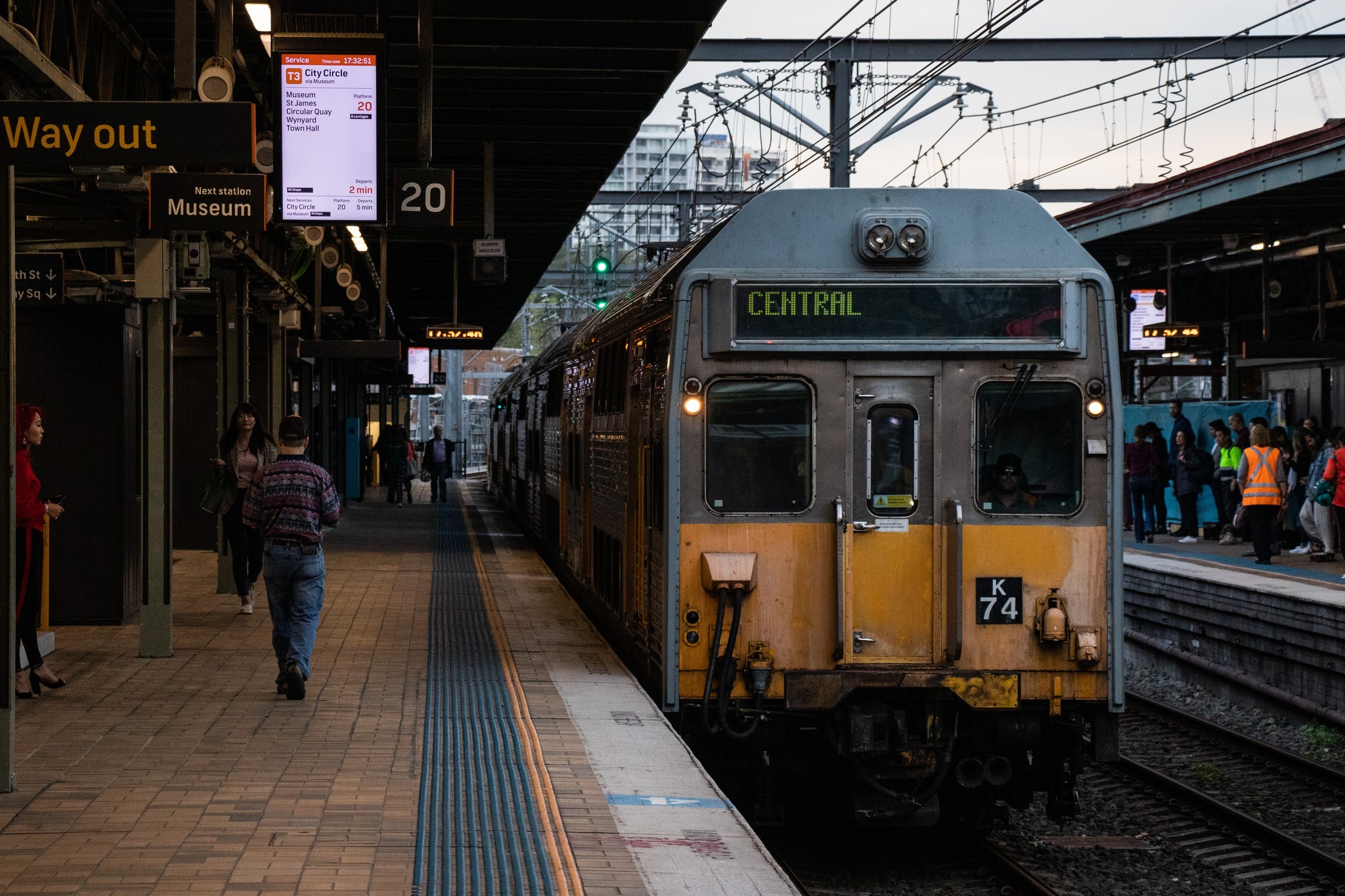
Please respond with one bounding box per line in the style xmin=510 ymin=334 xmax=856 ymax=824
xmin=44 ymin=504 xmax=48 ymax=514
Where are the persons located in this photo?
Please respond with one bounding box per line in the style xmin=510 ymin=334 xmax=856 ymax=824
xmin=1123 ymin=400 xmax=1345 ymax=580
xmin=211 ymin=402 xmax=277 ymax=614
xmin=421 ymin=425 xmax=455 ymax=503
xmin=14 ymin=400 xmax=67 ymax=699
xmin=979 ymin=452 xmax=1047 ymax=513
xmin=371 ymin=423 xmax=418 ymax=507
xmin=242 ymin=414 xmax=341 ymax=700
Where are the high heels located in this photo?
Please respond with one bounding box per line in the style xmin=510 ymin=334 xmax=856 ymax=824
xmin=29 ymin=668 xmax=67 ymax=695
xmin=15 ymin=689 xmax=38 ymax=699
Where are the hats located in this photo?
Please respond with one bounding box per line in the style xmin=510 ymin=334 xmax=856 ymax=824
xmin=278 ymin=415 xmax=309 ymax=440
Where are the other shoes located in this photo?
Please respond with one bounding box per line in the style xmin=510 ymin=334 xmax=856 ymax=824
xmin=286 ymin=664 xmax=305 ymax=700
xmin=407 ymin=492 xmax=413 ymax=505
xmin=248 ymin=583 xmax=256 ymax=607
xmin=1123 ymin=525 xmax=1198 ymax=543
xmin=397 ymin=503 xmax=402 ymax=508
xmin=241 ymin=604 xmax=253 ymax=614
xmin=277 ymin=682 xmax=288 ymax=693
xmin=1218 ymin=532 xmax=1343 ymax=565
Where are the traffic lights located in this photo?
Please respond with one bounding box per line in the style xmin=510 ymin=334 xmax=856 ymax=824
xmin=592 ymin=256 xmax=611 ymax=274
xmin=596 ymin=298 xmax=607 ymax=308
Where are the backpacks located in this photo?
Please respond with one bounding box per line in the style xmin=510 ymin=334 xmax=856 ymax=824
xmin=1185 ymin=447 xmax=1214 ymax=484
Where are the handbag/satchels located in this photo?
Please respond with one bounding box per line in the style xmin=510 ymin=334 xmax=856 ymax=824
xmin=1312 ymin=455 xmax=1337 ymax=506
xmin=405 ymin=458 xmax=419 ymax=478
xmin=1232 ymin=501 xmax=1248 ymax=528
xmin=200 ymin=471 xmax=227 ymax=514
xmin=420 ymin=471 xmax=431 ymax=483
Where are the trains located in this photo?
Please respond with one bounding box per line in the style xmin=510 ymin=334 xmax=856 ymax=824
xmin=488 ymin=186 xmax=1131 ymax=832
